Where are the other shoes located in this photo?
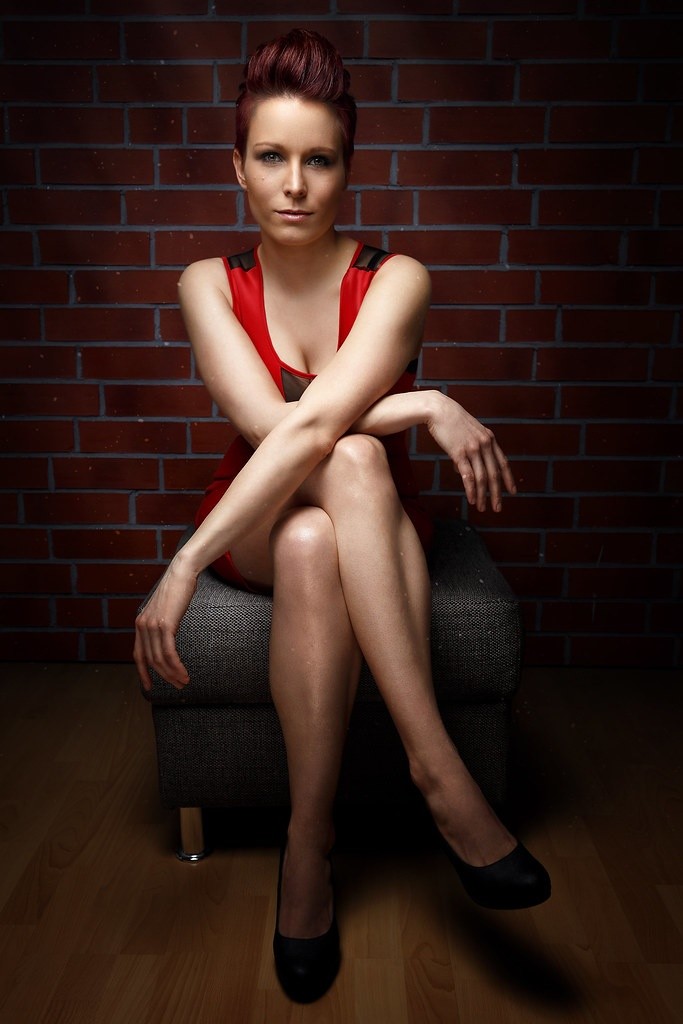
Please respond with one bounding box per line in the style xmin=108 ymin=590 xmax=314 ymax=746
xmin=272 ymin=836 xmax=342 ymax=1005
xmin=429 ymin=813 xmax=552 ymax=911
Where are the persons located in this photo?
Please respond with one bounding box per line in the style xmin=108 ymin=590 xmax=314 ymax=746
xmin=133 ymin=27 xmax=552 ymax=1003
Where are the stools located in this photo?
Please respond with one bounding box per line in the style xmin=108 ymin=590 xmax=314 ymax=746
xmin=138 ymin=515 xmax=524 ymax=862
